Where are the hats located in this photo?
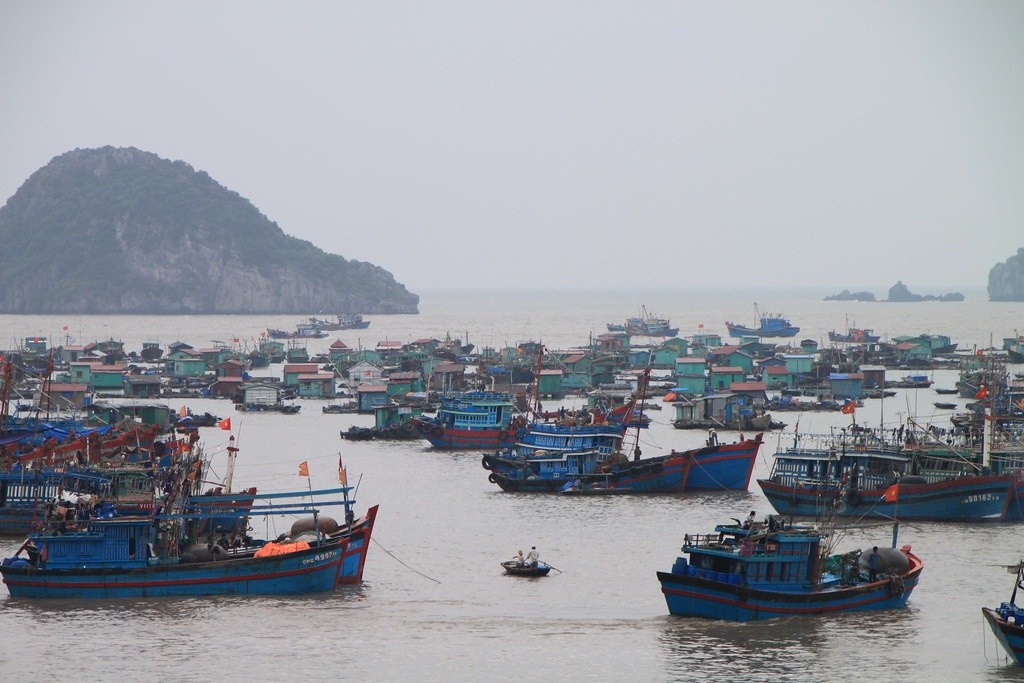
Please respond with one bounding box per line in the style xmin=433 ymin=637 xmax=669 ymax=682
xmin=532 ymin=544 xmax=536 ymax=549
xmin=518 ymin=550 xmax=523 ymax=555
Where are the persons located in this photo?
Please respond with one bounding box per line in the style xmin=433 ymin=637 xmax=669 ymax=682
xmin=514 ymin=550 xmax=524 ymax=567
xmin=526 ymin=546 xmax=539 ymax=567
xmin=634 ymin=447 xmax=641 ymax=460
xmin=218 ymin=533 xmax=229 ymax=550
xmin=709 ymin=428 xmax=718 ymax=446
xmin=41 ymin=543 xmax=48 ymax=570
xmin=743 ymin=511 xmax=756 ymax=530
xmin=545 ymin=406 xmax=567 ymax=422
xmin=868 ymin=546 xmax=880 ymax=583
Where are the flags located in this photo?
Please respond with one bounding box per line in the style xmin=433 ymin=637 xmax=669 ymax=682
xmin=299 ymin=461 xmax=309 ymax=476
xmin=63 ymin=325 xmax=68 ymax=329
xmin=977 ymin=351 xmax=982 ymax=355
xmin=218 ymin=417 xmax=230 ymax=429
xmin=975 ymin=386 xmax=987 ymax=398
xmin=886 ymin=483 xmax=899 ymax=502
xmin=842 ymin=400 xmax=855 ymax=413
xmin=339 ymin=468 xmax=347 ymax=485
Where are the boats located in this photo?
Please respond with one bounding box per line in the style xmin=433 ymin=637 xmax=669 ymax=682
xmin=267 ymin=317 xmax=329 ymax=339
xmin=725 ymin=301 xmax=800 ymax=338
xmin=931 ymin=335 xmax=959 ymax=353
xmin=982 ymin=554 xmax=1024 ymax=671
xmin=0 ymin=335 xmax=380 ymax=598
xmin=672 ymin=386 xmax=788 ymax=431
xmin=409 ymin=355 xmax=536 ymax=449
xmin=756 ymin=349 xmax=1024 ymax=524
xmin=308 ymin=301 xmax=371 ymax=329
xmin=483 ymin=407 xmax=765 ymax=495
xmin=607 ymin=305 xmax=681 ymax=337
xmin=501 ymin=562 xmax=550 ymax=575
xmin=829 ymin=312 xmax=880 ymax=343
xmin=514 ymin=338 xmax=653 ymax=463
xmin=559 ymin=472 xmax=630 ymax=494
xmin=432 ymin=328 xmax=475 ymax=354
xmin=656 ymin=435 xmax=925 ymax=618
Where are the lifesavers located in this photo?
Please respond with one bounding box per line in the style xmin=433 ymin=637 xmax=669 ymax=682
xmin=652 ymin=462 xmax=664 ymax=473
xmin=497 ymin=477 xmax=508 ymax=486
xmin=422 ymin=422 xmax=433 ymax=433
xmin=482 ymin=456 xmax=496 ymax=470
xmin=498 ymin=431 xmax=509 ymax=441
xmin=629 ymin=467 xmax=640 ymax=478
xmin=608 ymin=470 xmax=619 ymax=482
xmin=488 ymin=473 xmax=498 ymax=483
xmin=432 ymin=427 xmax=444 ymax=438
xmin=642 ymin=464 xmax=652 ymax=476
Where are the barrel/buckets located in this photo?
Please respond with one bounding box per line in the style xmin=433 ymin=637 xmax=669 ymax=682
xmin=995 ymin=602 xmax=1024 ymax=624
xmin=159 ymin=448 xmax=173 ymax=467
